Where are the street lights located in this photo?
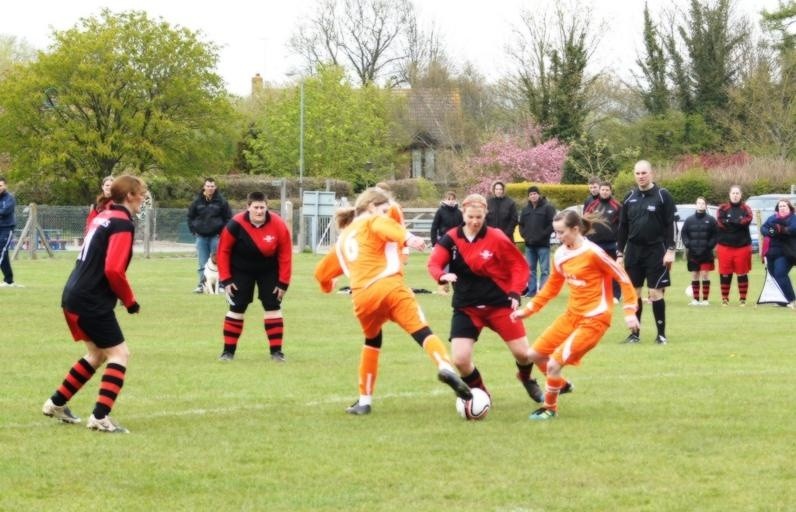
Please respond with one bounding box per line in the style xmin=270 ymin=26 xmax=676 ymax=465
xmin=285 ymin=66 xmax=304 ymax=211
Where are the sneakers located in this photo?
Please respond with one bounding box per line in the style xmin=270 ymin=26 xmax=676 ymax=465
xmin=271 ymin=351 xmax=284 ymax=363
xmin=612 ymin=297 xmax=619 ymax=305
xmin=86 ymin=414 xmax=129 ymax=434
xmin=439 ymin=285 xmax=449 ymax=294
xmin=623 ymin=334 xmax=640 ymax=343
xmin=689 ymin=299 xmax=796 ymax=311
xmin=219 ymin=287 xmax=224 ymax=292
xmin=655 ymin=335 xmax=667 ymax=344
xmin=193 ymin=285 xmax=203 ymax=294
xmin=0 ymin=281 xmax=16 ymax=287
xmin=346 ymin=369 xmax=573 ymax=420
xmin=217 ymin=351 xmax=234 ymax=362
xmin=41 ymin=399 xmax=82 ymax=424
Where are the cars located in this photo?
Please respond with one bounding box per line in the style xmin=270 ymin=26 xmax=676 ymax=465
xmin=743 ymin=194 xmax=796 ymax=250
xmin=673 ymin=202 xmax=721 ymax=249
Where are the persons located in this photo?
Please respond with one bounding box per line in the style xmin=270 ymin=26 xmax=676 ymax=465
xmin=519 ymin=186 xmax=555 ymax=298
xmin=430 ymin=190 xmax=463 ymax=293
xmin=681 ymin=186 xmax=796 ymax=309
xmin=43 ymin=176 xmax=145 ymax=432
xmin=187 ymin=177 xmax=232 ymax=294
xmin=526 ymin=209 xmax=642 ymax=419
xmin=218 ymin=192 xmax=292 ymax=362
xmin=427 ymin=194 xmax=544 ymax=405
xmin=583 ymin=160 xmax=679 ymax=343
xmin=484 ymin=181 xmax=518 ymax=242
xmin=0 ymin=178 xmax=16 ymax=285
xmin=314 ymin=182 xmax=474 ymax=415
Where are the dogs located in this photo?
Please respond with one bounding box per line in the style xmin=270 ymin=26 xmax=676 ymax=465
xmin=201 ymin=252 xmax=220 ymax=295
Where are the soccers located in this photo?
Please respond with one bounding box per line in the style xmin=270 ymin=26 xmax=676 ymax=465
xmin=455 ymin=388 xmax=491 ymax=420
